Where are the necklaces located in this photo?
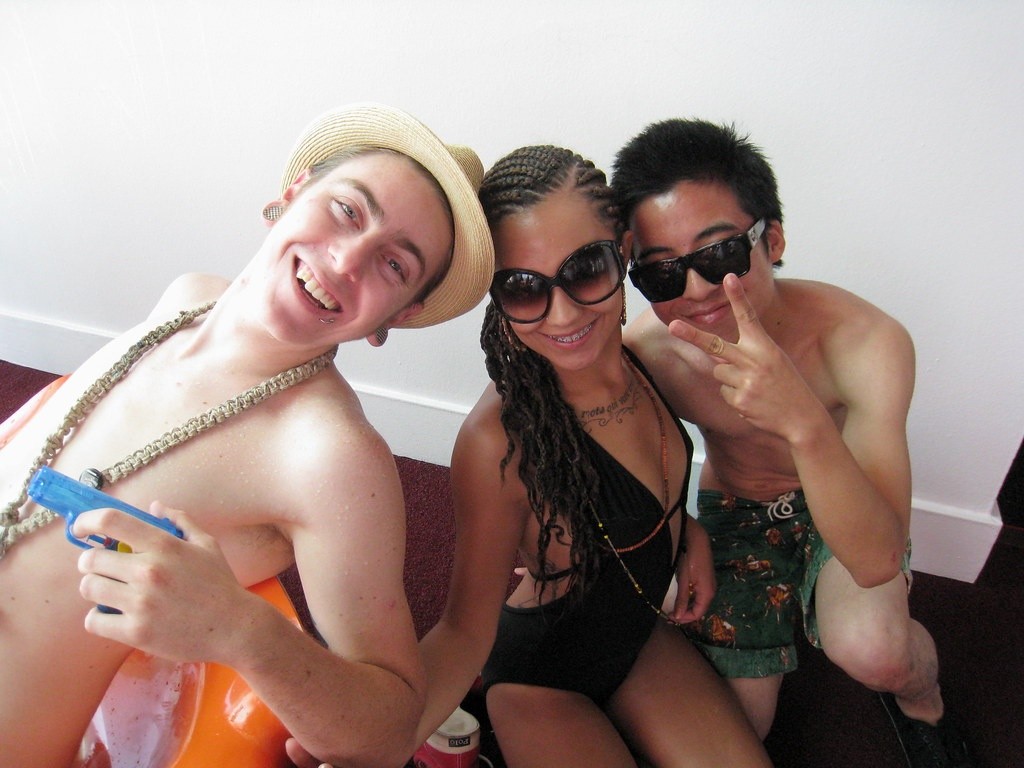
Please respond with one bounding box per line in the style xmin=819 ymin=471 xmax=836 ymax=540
xmin=546 ymin=344 xmax=694 ymax=628
xmin=0 ymin=299 xmax=339 ymax=563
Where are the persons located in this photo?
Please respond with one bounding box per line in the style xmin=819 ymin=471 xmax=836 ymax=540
xmin=513 ymin=119 xmax=977 ymax=768
xmin=285 ymin=145 xmax=786 ymax=768
xmin=0 ymin=103 xmax=495 ymax=768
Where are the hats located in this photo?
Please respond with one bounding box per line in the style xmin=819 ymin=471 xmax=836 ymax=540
xmin=280 ymin=104 xmax=495 ymax=329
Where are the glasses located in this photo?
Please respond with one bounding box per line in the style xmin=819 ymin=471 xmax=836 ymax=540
xmin=489 ymin=234 xmax=626 ymax=324
xmin=628 ymin=217 xmax=767 ymax=302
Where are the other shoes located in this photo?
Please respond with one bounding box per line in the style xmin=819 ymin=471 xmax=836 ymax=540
xmin=878 ymin=691 xmax=986 ymax=768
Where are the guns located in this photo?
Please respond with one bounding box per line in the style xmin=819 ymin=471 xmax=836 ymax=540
xmin=26 ymin=466 xmax=185 ymax=616
